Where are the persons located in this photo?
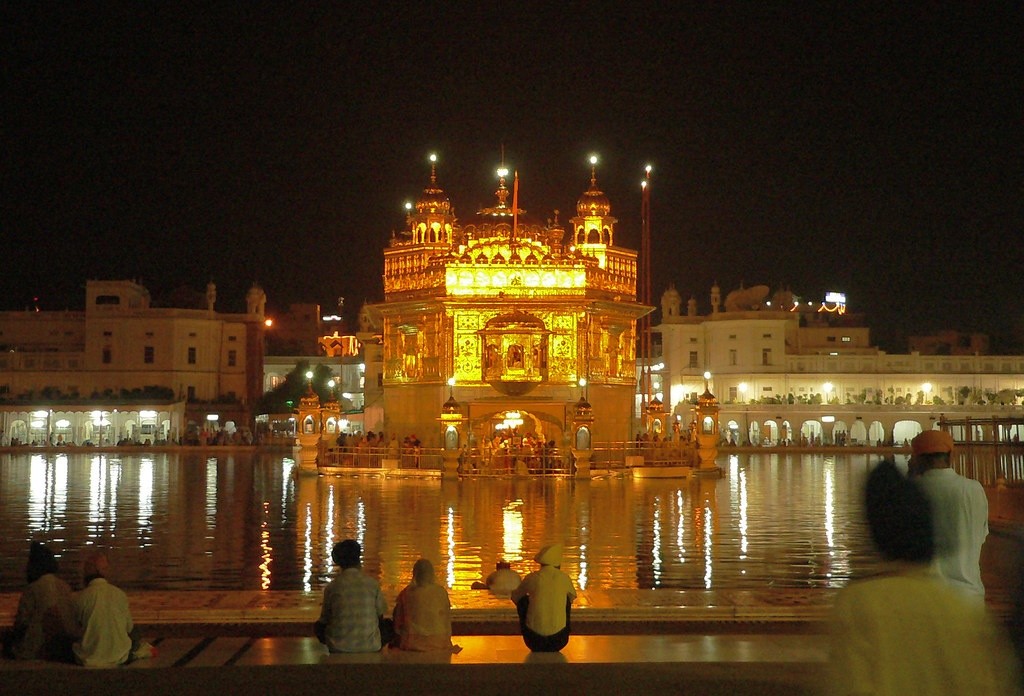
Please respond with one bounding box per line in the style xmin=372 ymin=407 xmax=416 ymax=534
xmin=877 ymin=438 xmax=882 ymax=447
xmin=776 ymin=429 xmax=846 ymax=447
xmin=314 ymin=540 xmax=451 ymax=654
xmin=334 ymin=430 xmax=422 ymax=470
xmin=908 ymin=430 xmax=990 ymax=603
xmin=511 ymin=543 xmax=577 ymax=652
xmin=822 ymin=460 xmax=1024 ymax=696
xmin=246 ymin=427 xmax=290 ymax=445
xmin=903 ymin=439 xmax=909 ymax=448
xmin=471 ymin=561 xmax=523 ymax=590
xmin=720 ymin=438 xmax=736 ymax=447
xmin=532 ymin=439 xmax=562 ymax=474
xmin=5 ymin=541 xmax=132 ymax=669
xmin=634 ymin=432 xmax=700 ymax=448
xmin=0 ymin=430 xmax=168 ymax=447
xmin=188 ymin=430 xmax=196 ymax=446
xmin=199 ymin=427 xmax=242 ymax=446
